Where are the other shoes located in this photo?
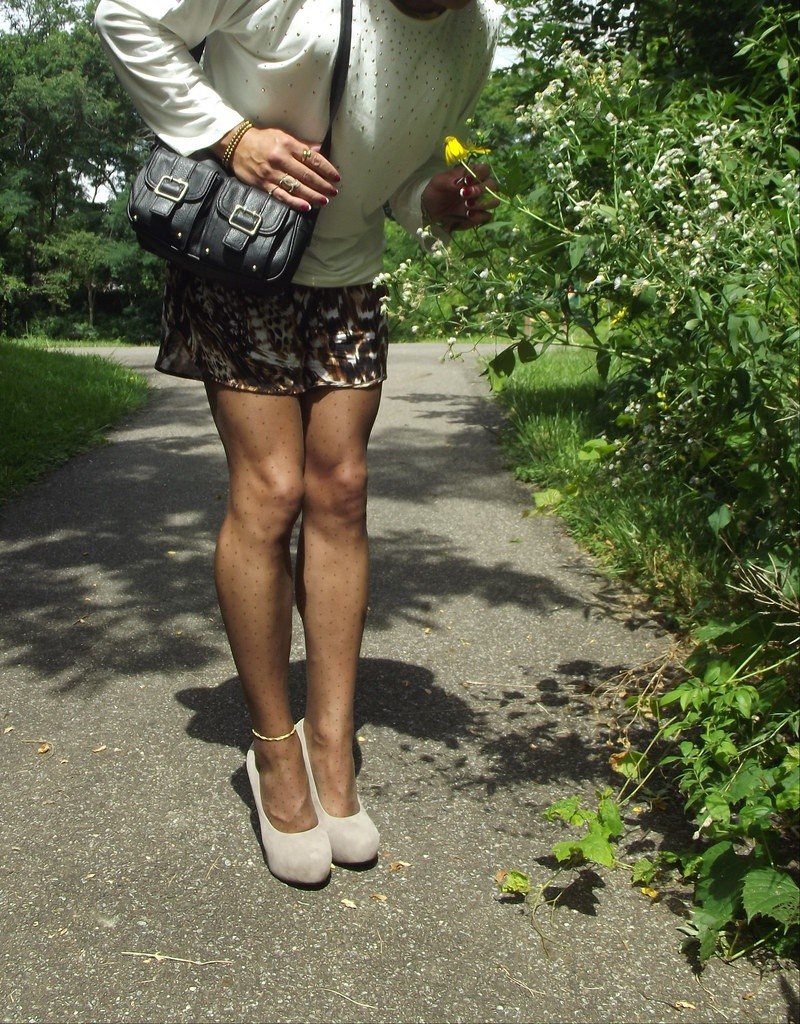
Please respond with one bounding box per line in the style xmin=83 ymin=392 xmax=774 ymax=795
xmin=246 ymin=742 xmax=333 ymax=886
xmin=295 ymin=718 xmax=379 ymax=865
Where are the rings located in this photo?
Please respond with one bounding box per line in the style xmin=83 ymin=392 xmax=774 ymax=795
xmin=300 ymin=147 xmax=312 ymax=162
xmin=279 ymin=173 xmax=299 ymax=193
xmin=267 ymin=186 xmax=279 ymax=195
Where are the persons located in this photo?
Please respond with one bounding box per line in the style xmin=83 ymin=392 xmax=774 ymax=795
xmin=93 ymin=0 xmax=501 ymax=887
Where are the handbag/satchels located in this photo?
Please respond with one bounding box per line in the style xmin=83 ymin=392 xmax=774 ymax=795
xmin=126 ymin=0 xmax=353 ymax=292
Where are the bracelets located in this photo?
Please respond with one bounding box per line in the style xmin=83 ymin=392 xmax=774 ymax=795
xmin=220 ymin=120 xmax=254 ymax=170
